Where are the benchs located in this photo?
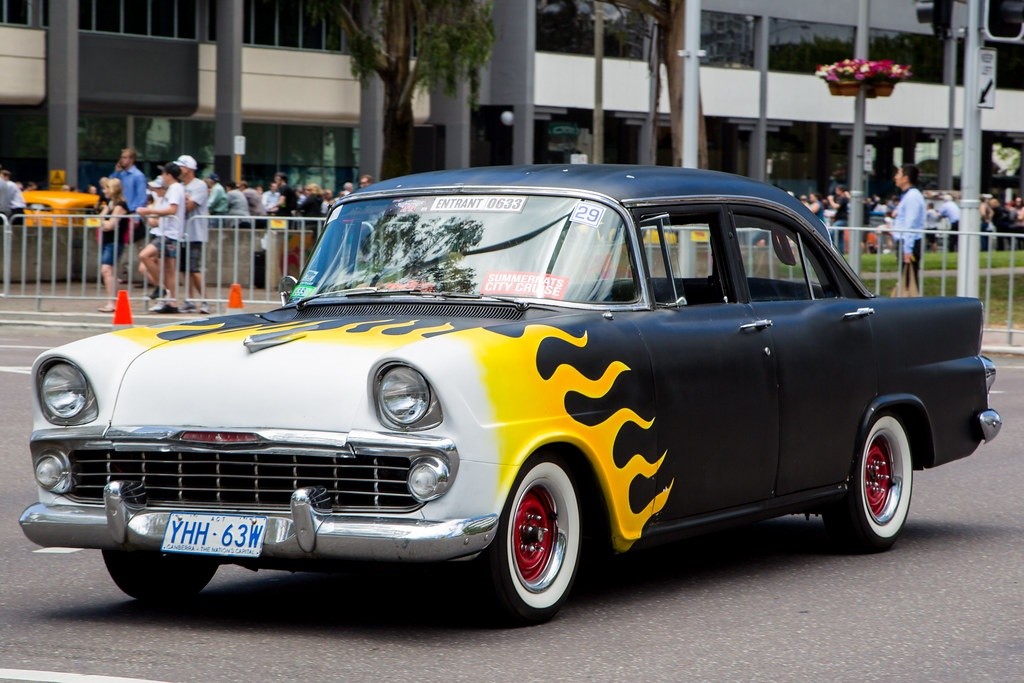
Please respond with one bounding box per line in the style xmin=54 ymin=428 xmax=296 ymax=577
xmin=611 ymin=273 xmax=833 ymax=308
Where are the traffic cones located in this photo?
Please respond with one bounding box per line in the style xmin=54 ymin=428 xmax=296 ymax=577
xmin=224 ymin=283 xmax=248 ymax=315
xmin=113 ymin=288 xmax=134 ymax=326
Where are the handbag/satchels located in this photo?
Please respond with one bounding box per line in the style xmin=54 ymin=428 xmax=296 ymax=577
xmin=890 ymin=261 xmax=918 ymax=297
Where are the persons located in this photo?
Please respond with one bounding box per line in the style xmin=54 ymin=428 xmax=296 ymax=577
xmin=203 ymin=171 xmax=375 ymax=230
xmin=876 ymin=165 xmax=926 ymax=294
xmin=784 ymin=184 xmax=850 ymax=256
xmin=939 ymin=195 xmax=959 ymax=252
xmin=925 ymin=203 xmax=940 ymax=251
xmin=980 ymin=196 xmax=1024 ymax=251
xmin=136 ymin=155 xmax=211 ymax=315
xmin=109 ymin=149 xmax=148 ymax=282
xmin=861 ymin=191 xmax=899 ymax=254
xmin=94 ymin=175 xmax=130 ymax=311
xmin=0 ymin=170 xmax=109 ymax=225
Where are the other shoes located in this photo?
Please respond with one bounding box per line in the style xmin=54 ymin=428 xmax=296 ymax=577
xmin=156 ymin=305 xmax=178 ymax=314
xmin=98 ymin=305 xmax=115 ymax=312
xmin=149 ymin=301 xmax=165 ymax=311
xmin=149 ymin=287 xmax=167 ymax=298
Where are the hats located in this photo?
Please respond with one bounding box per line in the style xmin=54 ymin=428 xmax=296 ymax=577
xmin=148 ymin=175 xmax=165 ymax=188
xmin=236 ymin=181 xmax=248 ymax=188
xmin=158 ymin=163 xmax=181 ymax=179
xmin=173 ymin=155 xmax=198 ymax=170
xmin=209 ymin=174 xmax=220 ymax=181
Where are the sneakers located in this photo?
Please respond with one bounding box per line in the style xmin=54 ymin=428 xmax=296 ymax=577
xmin=177 ymin=301 xmax=196 ymax=312
xmin=199 ymin=302 xmax=210 ymax=314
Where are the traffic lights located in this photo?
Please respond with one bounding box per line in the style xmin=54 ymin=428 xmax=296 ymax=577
xmin=915 ymin=1 xmax=949 ymax=39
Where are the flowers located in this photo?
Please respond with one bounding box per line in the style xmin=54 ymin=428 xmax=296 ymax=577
xmin=813 ymin=58 xmax=912 ymax=83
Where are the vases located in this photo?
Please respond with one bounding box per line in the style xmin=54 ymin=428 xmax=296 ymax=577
xmin=829 ymin=81 xmax=897 ymax=98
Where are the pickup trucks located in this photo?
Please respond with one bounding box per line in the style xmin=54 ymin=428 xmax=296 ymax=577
xmin=19 ymin=163 xmax=1001 ymax=625
